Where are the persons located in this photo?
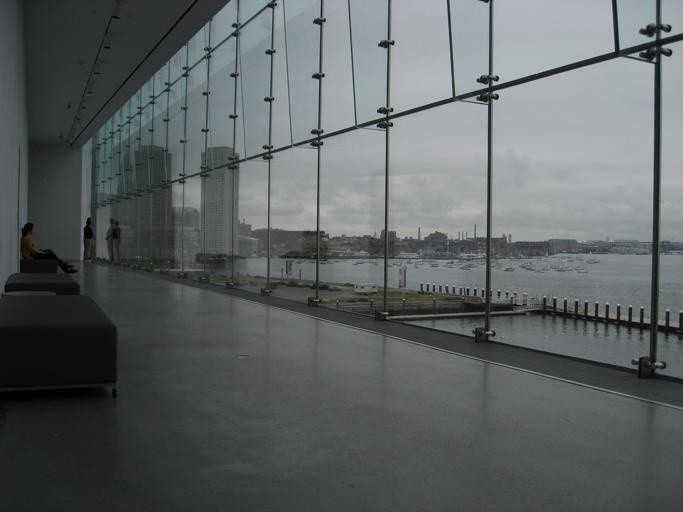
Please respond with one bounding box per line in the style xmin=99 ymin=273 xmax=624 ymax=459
xmin=86 ymin=216 xmax=97 ymax=248
xmin=84 ymin=220 xmax=92 ymax=262
xmin=20 ymin=222 xmax=78 ymax=273
xmin=104 ymin=218 xmax=114 ymax=260
xmin=110 ymin=220 xmax=121 ymax=263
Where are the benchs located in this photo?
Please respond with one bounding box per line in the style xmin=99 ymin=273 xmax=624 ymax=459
xmin=2 ymin=255 xmax=118 ymax=400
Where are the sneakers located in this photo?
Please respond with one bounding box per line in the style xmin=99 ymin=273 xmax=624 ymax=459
xmin=64 ymin=264 xmax=78 ymax=274
